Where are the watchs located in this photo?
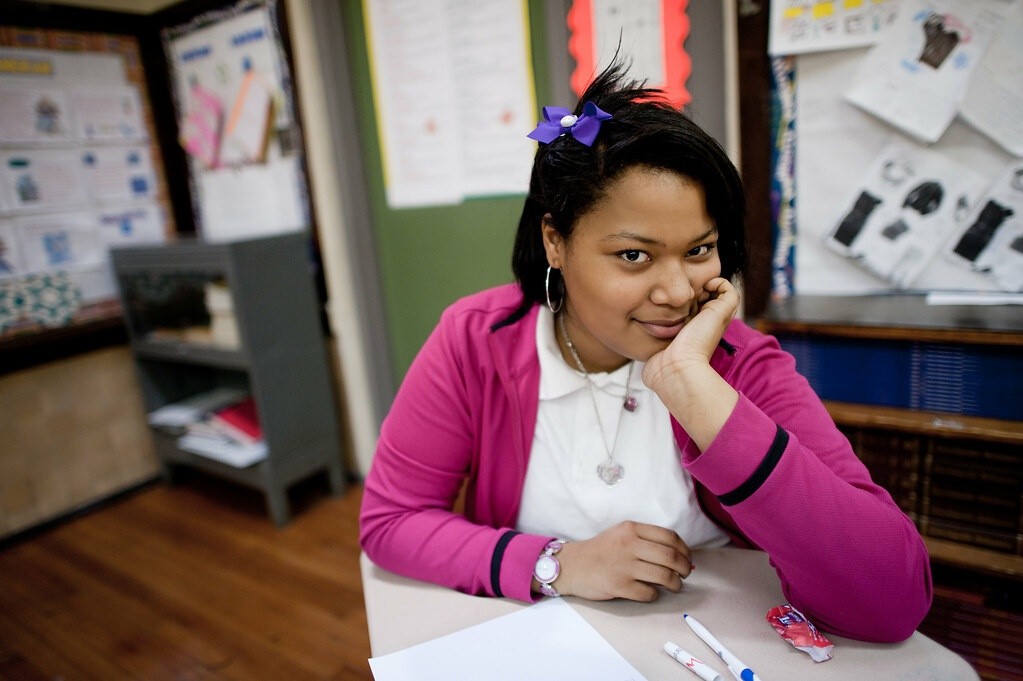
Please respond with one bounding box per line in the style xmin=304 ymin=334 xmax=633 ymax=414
xmin=532 ymin=538 xmax=570 ymax=599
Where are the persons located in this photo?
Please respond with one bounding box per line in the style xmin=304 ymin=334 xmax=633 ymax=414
xmin=356 ymin=54 xmax=934 ymax=643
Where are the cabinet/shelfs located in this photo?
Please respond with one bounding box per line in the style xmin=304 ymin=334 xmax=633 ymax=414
xmin=110 ymin=231 xmax=345 ymax=527
xmin=740 ymin=291 xmax=1023 ymax=681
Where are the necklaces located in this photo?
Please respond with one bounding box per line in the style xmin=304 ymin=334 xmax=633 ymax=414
xmin=555 ymin=308 xmax=638 ymax=485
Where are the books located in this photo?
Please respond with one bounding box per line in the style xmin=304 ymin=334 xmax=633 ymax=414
xmin=146 ymin=274 xmax=241 ymax=352
xmin=146 ymin=385 xmax=267 ymax=471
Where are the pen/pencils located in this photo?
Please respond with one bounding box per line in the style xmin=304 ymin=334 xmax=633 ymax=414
xmin=664 ymin=641 xmax=725 ymax=681
xmin=684 ymin=613 xmax=761 ymax=681
